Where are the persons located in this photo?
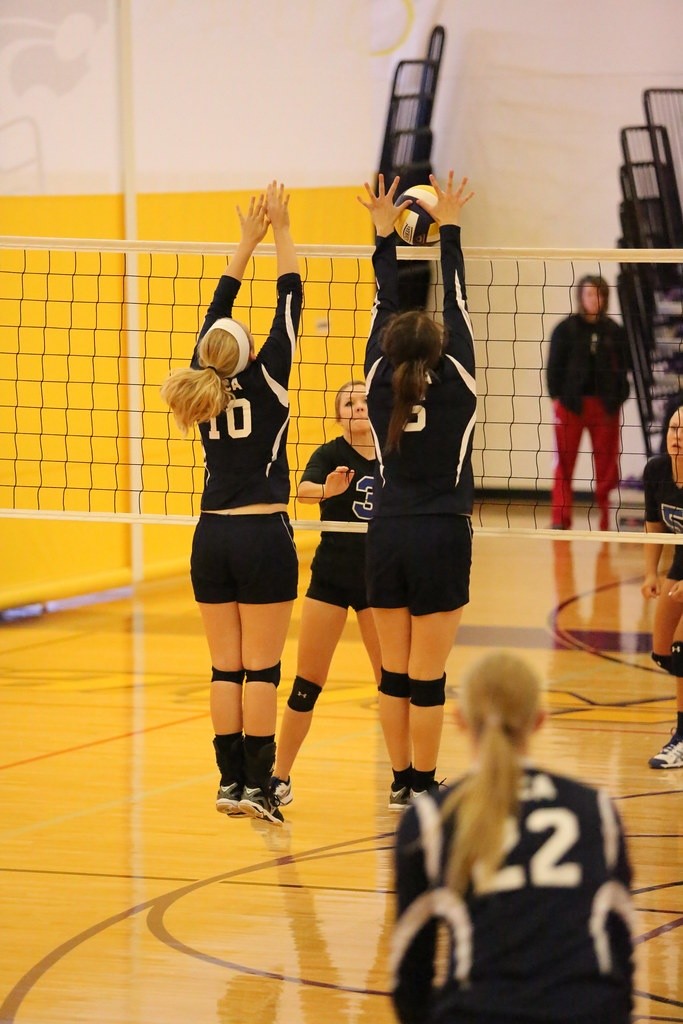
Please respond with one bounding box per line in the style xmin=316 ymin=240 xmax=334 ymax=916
xmin=389 ymin=651 xmax=638 ymax=1024
xmin=160 ymin=181 xmax=304 ymax=826
xmin=271 ymin=381 xmax=381 ymax=805
xmin=639 ymin=399 xmax=683 ymax=769
xmin=545 ymin=275 xmax=633 ymax=531
xmin=362 ymin=172 xmax=478 ymax=808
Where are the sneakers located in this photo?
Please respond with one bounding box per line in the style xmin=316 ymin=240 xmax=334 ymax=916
xmin=237 ymin=785 xmax=285 ymax=827
xmin=388 ymin=777 xmax=438 ymax=810
xmin=214 ymin=781 xmax=247 ymax=818
xmin=268 ymin=774 xmax=293 ymax=806
xmin=647 ymin=735 xmax=683 ymax=768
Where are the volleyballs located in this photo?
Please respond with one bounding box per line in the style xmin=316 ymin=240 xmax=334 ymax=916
xmin=391 ymin=184 xmax=442 ymax=245
